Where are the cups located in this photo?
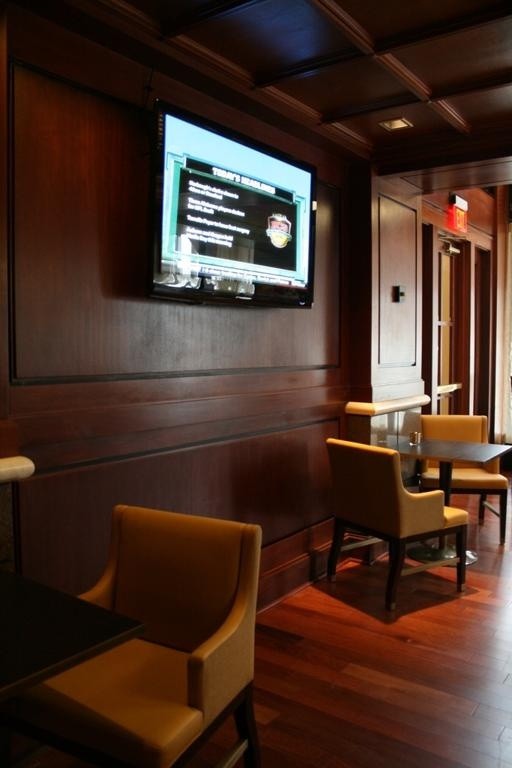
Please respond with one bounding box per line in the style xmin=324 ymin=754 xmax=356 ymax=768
xmin=408 ymin=431 xmax=421 ymax=447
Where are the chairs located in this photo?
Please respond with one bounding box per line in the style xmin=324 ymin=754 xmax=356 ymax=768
xmin=0 ymin=504 xmax=263 ymax=768
xmin=325 ymin=415 xmax=512 ymax=611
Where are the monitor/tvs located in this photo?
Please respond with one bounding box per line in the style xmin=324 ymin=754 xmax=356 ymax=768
xmin=145 ymin=96 xmax=318 ymax=309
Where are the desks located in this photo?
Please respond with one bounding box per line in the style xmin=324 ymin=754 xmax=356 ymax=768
xmin=0 ymin=569 xmax=144 ymax=701
